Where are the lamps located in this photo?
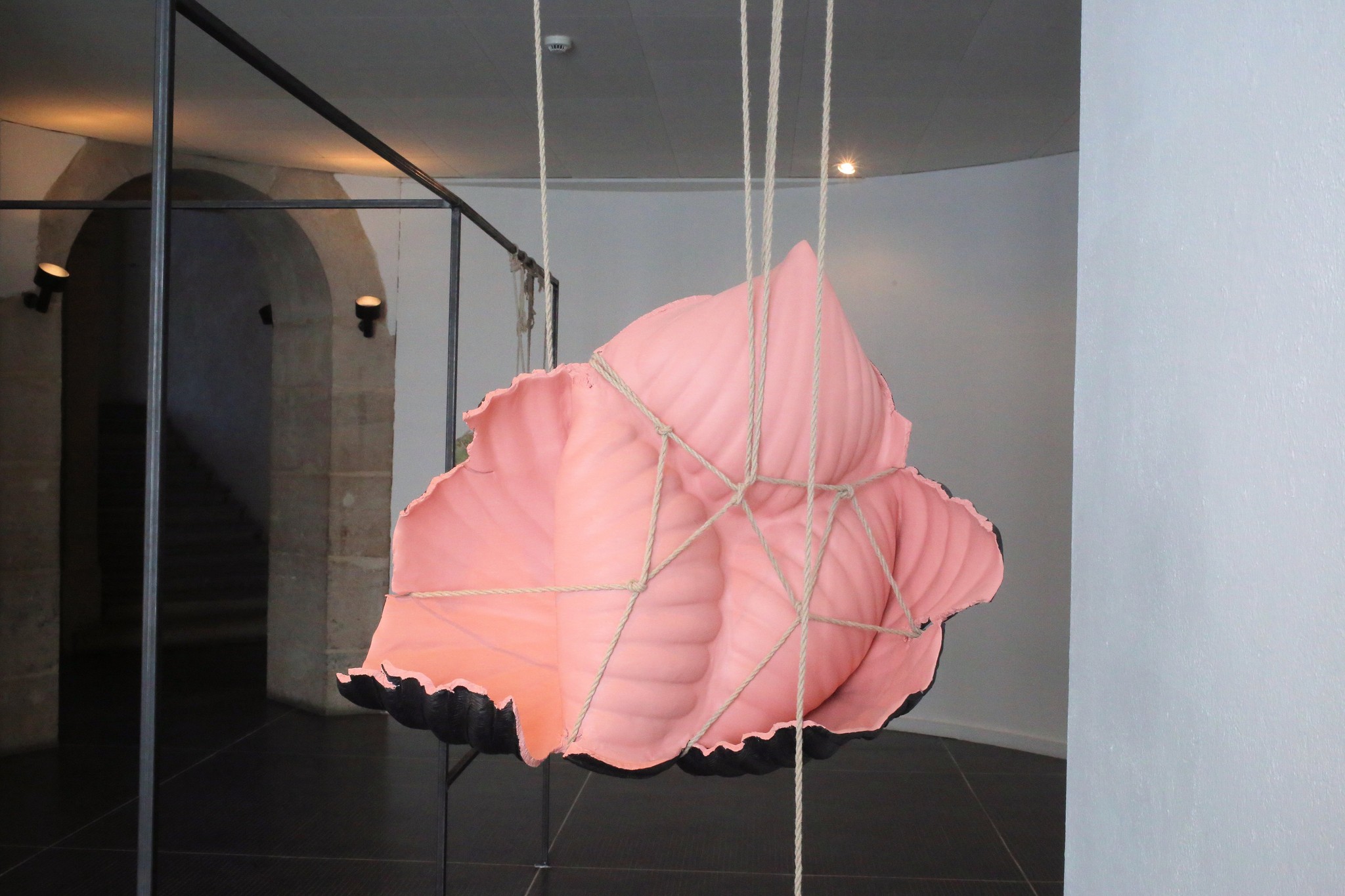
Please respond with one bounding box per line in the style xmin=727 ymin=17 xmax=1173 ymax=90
xmin=352 ymin=293 xmax=383 ymax=338
xmin=255 ymin=303 xmax=276 ymax=329
xmin=16 ymin=260 xmax=72 ymax=310
